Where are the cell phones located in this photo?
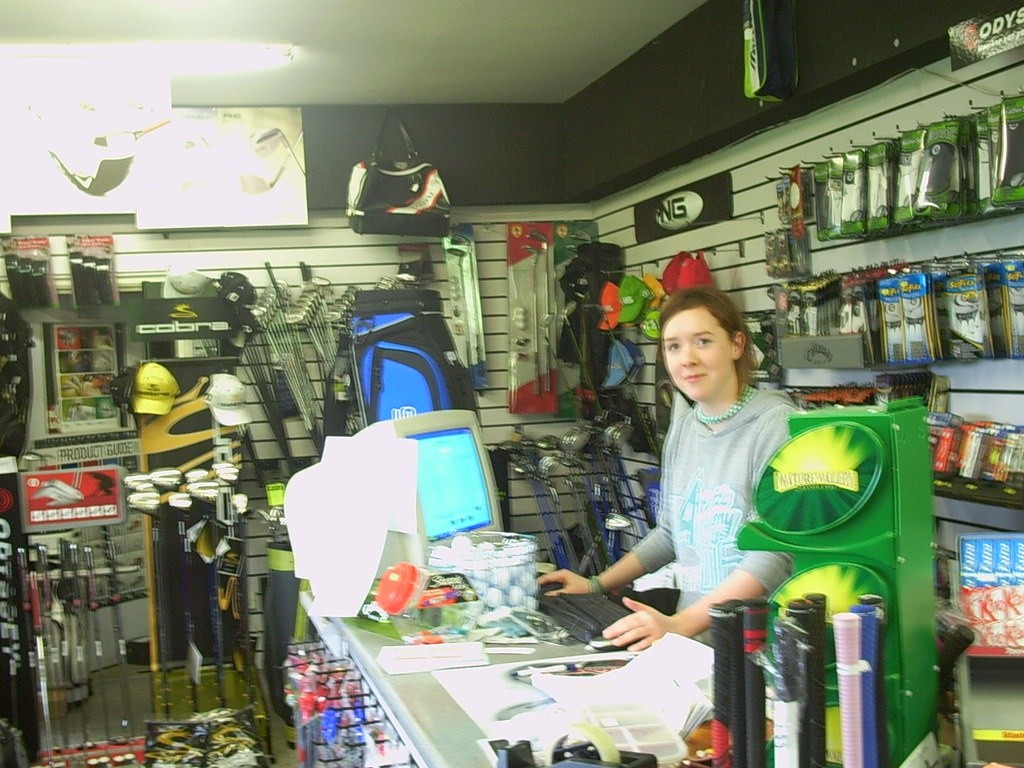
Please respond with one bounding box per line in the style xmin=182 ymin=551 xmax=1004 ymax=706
xmin=588 ymin=634 xmax=639 ymax=652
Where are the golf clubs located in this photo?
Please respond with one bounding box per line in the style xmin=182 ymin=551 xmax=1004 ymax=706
xmin=189 ymin=184 xmax=657 ymax=589
xmin=122 ymin=461 xmax=251 ymax=714
xmin=13 ymin=527 xmax=134 ymax=753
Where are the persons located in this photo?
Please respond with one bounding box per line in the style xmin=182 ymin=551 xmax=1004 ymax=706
xmin=535 ymin=285 xmax=799 ymax=658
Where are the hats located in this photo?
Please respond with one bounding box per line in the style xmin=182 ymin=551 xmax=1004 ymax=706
xmin=131 ymin=363 xmax=179 ymax=416
xmin=594 ymin=252 xmax=717 ymax=389
xmin=202 ymin=373 xmax=254 ymax=427
xmin=217 ymin=270 xmax=263 ymax=348
xmin=167 ymin=262 xmax=221 ymax=294
xmin=108 ymin=372 xmax=133 ymax=411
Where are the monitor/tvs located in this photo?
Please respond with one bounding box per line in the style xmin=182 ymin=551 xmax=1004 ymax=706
xmin=350 ymin=408 xmax=502 ymax=578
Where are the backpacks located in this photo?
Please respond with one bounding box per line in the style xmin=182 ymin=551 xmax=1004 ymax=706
xmin=345 ymin=292 xmax=476 ymax=424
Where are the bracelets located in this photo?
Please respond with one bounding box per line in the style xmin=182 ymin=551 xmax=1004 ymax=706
xmin=590 ymin=575 xmax=607 ymax=595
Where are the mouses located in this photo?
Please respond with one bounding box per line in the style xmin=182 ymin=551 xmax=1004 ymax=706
xmin=538 ymin=580 xmax=565 ymax=595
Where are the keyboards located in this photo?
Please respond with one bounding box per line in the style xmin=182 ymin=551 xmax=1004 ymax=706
xmin=536 ymin=592 xmax=635 ymax=645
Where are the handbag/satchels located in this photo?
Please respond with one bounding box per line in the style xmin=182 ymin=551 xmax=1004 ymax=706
xmin=346 ymin=106 xmax=452 ymax=236
